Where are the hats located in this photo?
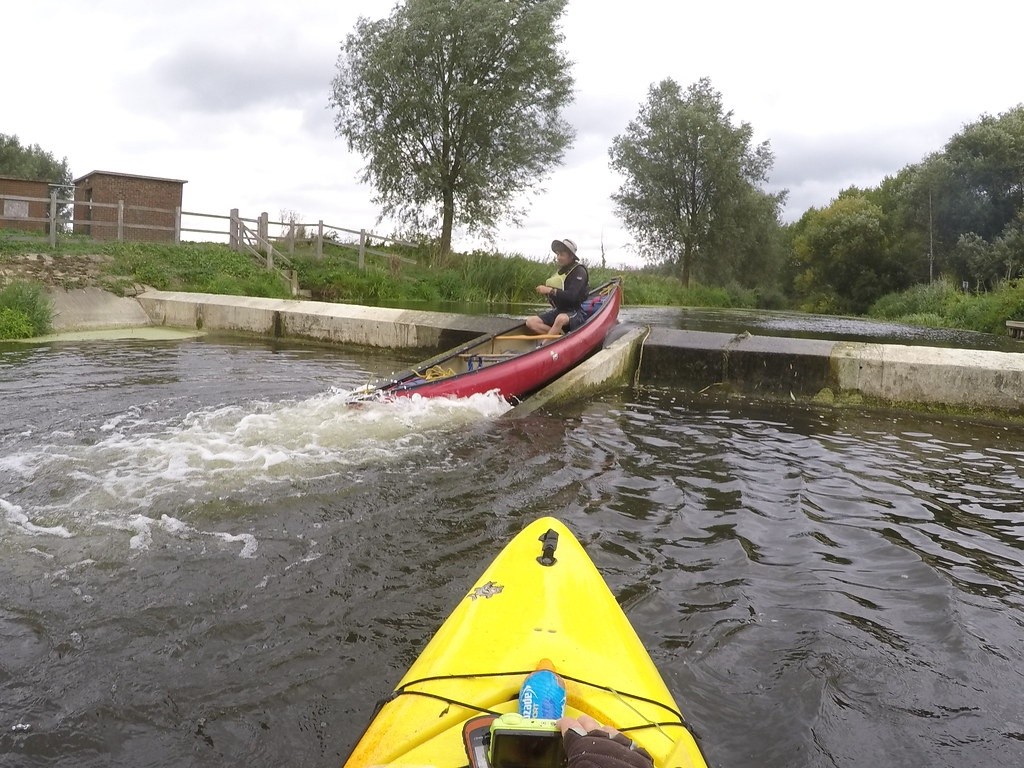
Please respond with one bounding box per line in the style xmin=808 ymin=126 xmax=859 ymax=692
xmin=551 ymin=238 xmax=581 ymax=262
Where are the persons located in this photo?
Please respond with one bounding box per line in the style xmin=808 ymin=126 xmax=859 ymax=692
xmin=526 ymin=239 xmax=589 ymax=349
xmin=555 ymin=715 xmax=655 ymax=768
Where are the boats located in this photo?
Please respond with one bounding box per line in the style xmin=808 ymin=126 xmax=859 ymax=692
xmin=350 ymin=275 xmax=622 ymax=409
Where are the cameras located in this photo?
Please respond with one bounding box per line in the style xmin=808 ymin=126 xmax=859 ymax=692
xmin=487 ymin=712 xmax=569 ymax=768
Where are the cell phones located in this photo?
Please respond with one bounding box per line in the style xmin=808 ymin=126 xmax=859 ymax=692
xmin=462 ymin=715 xmax=500 ymax=768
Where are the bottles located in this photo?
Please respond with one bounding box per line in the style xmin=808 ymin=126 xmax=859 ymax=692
xmin=519 ymin=658 xmax=566 ymax=720
